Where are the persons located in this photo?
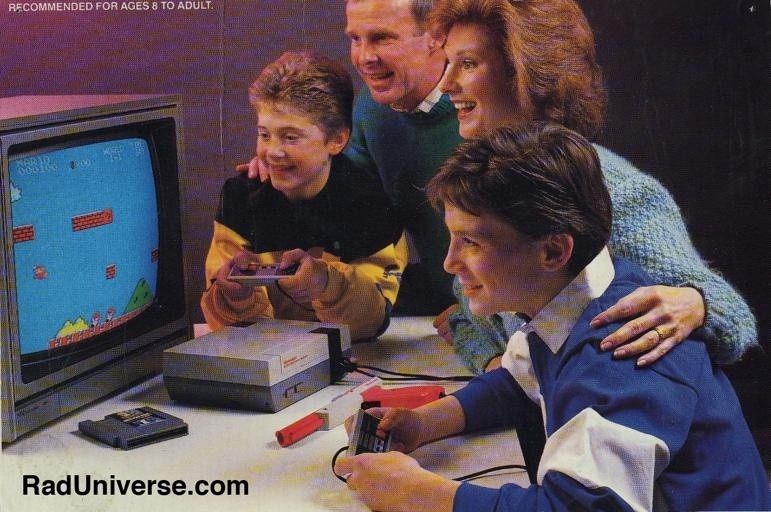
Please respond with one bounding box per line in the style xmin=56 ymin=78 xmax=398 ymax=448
xmin=335 ymin=116 xmax=771 ymax=511
xmin=199 ymin=51 xmax=422 ymax=343
xmin=234 ymin=0 xmax=491 ymax=315
xmin=271 ymin=374 xmax=450 ymax=449
xmin=425 ymin=0 xmax=759 ymax=382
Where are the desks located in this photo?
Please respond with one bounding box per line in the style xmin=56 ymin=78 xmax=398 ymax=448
xmin=2 ymin=315 xmax=531 ymax=511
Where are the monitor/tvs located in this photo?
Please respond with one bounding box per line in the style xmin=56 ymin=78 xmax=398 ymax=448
xmin=0 ymin=93 xmax=195 ymax=445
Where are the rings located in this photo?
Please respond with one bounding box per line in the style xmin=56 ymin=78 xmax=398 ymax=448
xmin=655 ymin=326 xmax=666 ymax=341
